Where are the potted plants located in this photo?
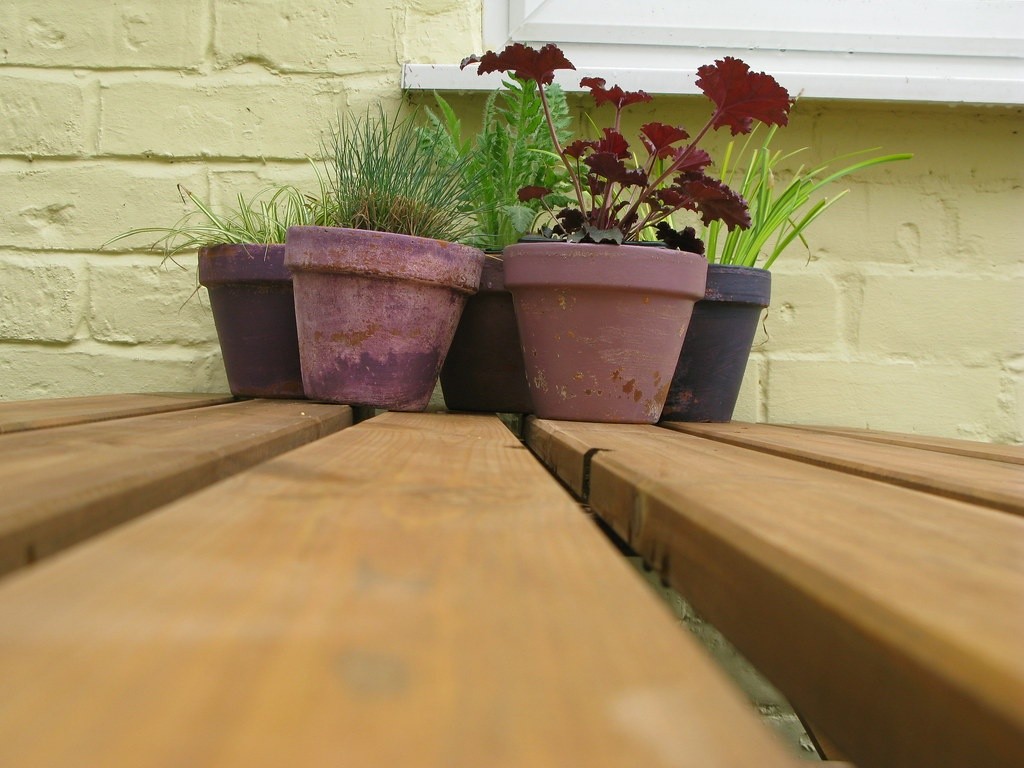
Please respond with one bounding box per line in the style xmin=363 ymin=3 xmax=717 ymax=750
xmin=94 ymin=39 xmax=915 ymax=427
